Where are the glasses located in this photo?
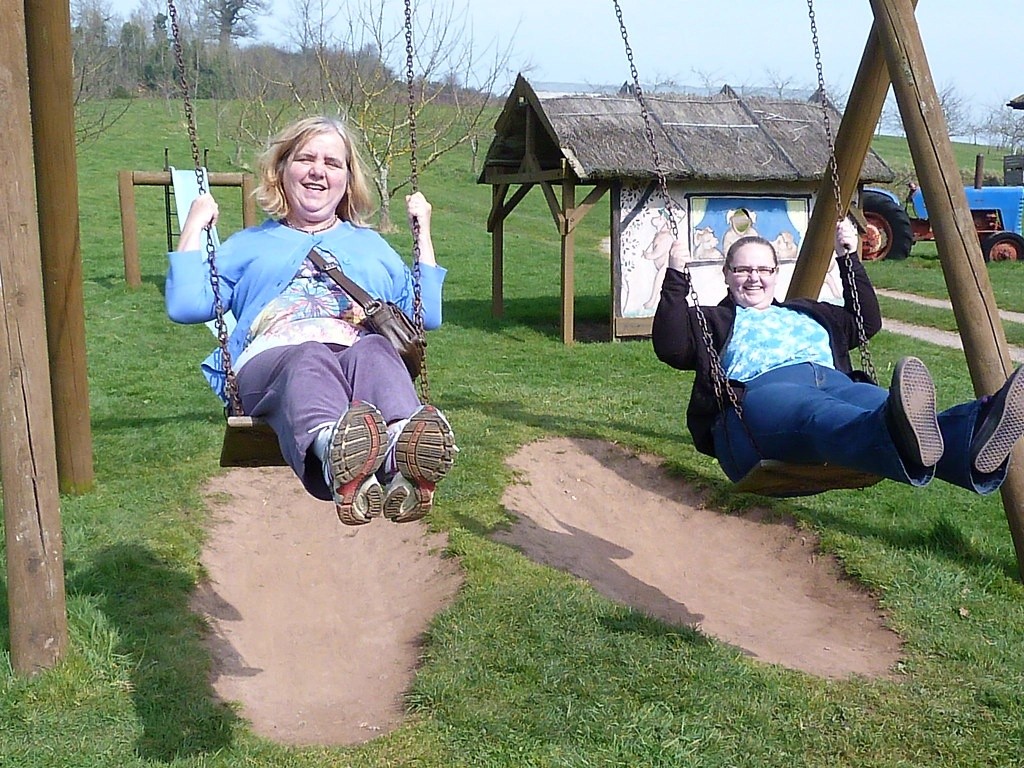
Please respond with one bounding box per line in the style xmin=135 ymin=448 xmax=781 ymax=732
xmin=727 ymin=262 xmax=776 ymax=277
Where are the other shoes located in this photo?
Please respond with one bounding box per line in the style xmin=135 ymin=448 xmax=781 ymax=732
xmin=887 ymin=356 xmax=944 ymax=468
xmin=969 ymin=364 xmax=1024 ymax=475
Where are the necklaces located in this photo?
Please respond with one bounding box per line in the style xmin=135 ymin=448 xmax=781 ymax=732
xmin=281 ymin=215 xmax=337 ymax=234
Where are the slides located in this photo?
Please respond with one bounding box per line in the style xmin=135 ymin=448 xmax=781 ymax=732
xmin=167 ymin=164 xmax=238 ymax=341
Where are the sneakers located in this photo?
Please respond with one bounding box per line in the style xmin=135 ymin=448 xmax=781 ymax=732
xmin=381 ymin=404 xmax=455 ymax=523
xmin=307 ymin=400 xmax=389 ymax=526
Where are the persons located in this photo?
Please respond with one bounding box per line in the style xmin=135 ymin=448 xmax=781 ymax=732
xmin=165 ymin=115 xmax=460 ymax=526
xmin=652 ymin=222 xmax=1024 ymax=496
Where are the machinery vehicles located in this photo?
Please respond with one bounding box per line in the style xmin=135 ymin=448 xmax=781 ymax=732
xmin=859 ymin=152 xmax=1024 ymax=263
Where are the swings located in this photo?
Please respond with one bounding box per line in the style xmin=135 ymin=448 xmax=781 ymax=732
xmin=608 ymin=0 xmax=892 ymax=499
xmin=158 ymin=0 xmax=434 ymax=469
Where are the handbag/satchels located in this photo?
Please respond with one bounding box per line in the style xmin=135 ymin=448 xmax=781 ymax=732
xmin=368 ymin=300 xmax=424 ymax=379
